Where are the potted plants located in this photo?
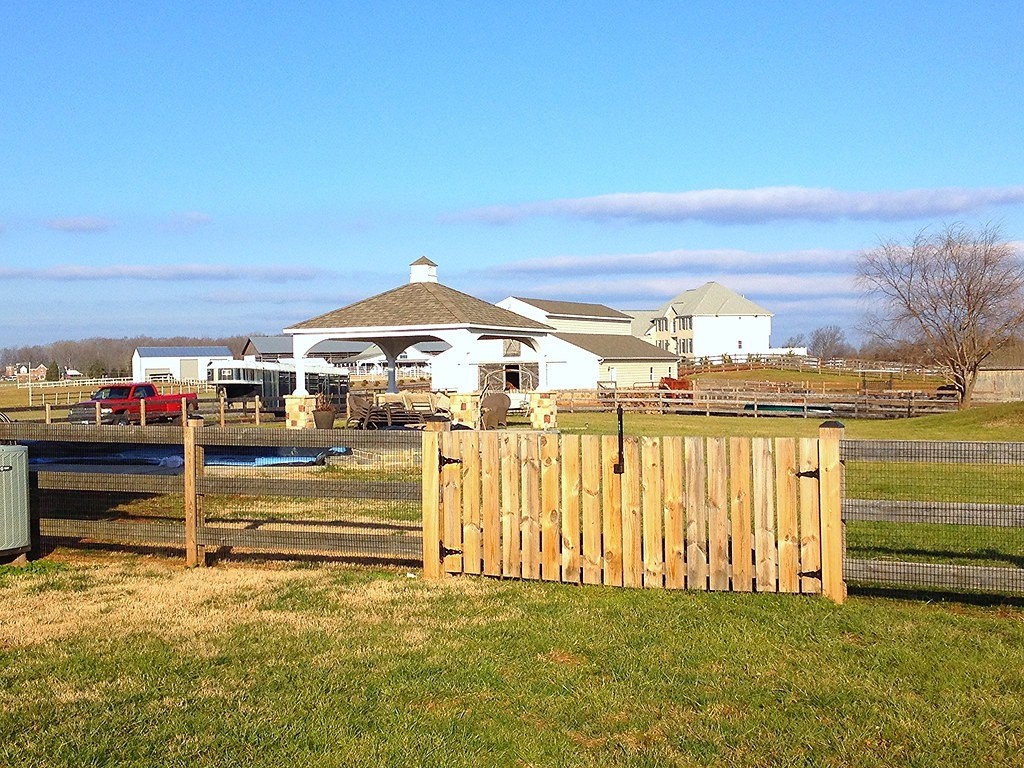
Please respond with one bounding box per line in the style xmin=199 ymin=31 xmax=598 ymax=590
xmin=311 ymin=392 xmax=337 ymax=429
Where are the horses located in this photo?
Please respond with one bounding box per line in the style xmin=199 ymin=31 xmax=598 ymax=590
xmin=658 ymin=376 xmax=694 ymax=403
xmin=937 ymin=385 xmax=963 ymax=400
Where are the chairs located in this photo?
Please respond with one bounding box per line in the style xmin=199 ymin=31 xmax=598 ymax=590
xmin=346 ymin=393 xmax=511 ymax=433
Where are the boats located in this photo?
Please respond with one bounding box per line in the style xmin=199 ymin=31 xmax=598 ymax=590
xmin=743 ymin=394 xmax=836 ymax=419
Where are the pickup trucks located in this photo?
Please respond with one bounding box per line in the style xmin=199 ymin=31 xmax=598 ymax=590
xmin=68 ymin=383 xmax=199 ymax=428
